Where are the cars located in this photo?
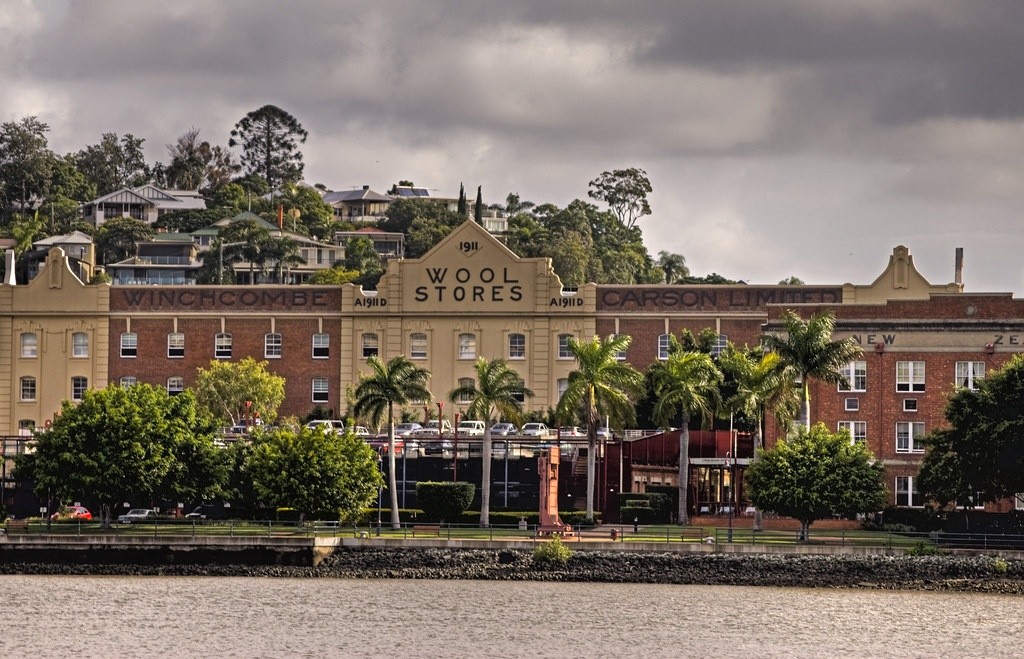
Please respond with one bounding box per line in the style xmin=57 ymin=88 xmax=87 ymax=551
xmin=598 ymin=427 xmax=618 ymax=441
xmin=701 ymin=505 xmax=709 ymax=515
xmin=561 ymin=426 xmax=588 ymax=436
xmin=336 ymin=425 xmax=369 ymax=435
xmin=655 ymin=426 xmax=679 ymax=435
xmin=393 ymin=423 xmax=423 ymax=436
xmin=745 ymin=505 xmax=756 ymax=518
xmin=520 ymin=423 xmax=550 ymax=439
xmin=719 ymin=506 xmax=734 ymax=516
xmin=119 ymin=509 xmax=158 ymax=523
xmin=489 ymin=423 xmax=519 ymax=437
xmin=51 ymin=506 xmax=93 ymax=521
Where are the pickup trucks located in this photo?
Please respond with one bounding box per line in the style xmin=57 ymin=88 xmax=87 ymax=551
xmin=305 ymin=420 xmax=345 ymax=437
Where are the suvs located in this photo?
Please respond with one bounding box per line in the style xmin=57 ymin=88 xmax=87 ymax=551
xmin=413 ymin=420 xmax=452 ymax=436
xmin=184 ymin=503 xmax=228 ymax=520
xmin=230 ymin=417 xmax=264 ymax=434
xmin=452 ymin=420 xmax=486 ymax=437
